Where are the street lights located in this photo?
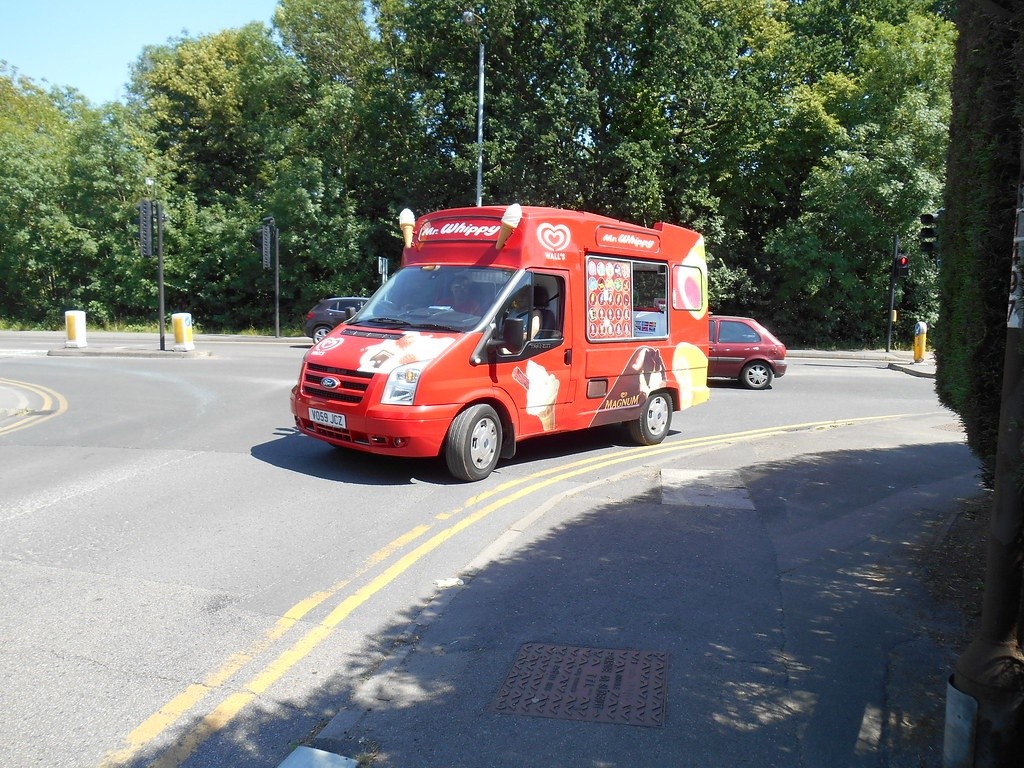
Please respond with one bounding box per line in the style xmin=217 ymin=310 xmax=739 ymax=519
xmin=462 ymin=11 xmax=487 ymax=208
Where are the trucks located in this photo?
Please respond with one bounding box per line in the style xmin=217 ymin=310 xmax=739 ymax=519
xmin=290 ymin=203 xmax=709 ymax=484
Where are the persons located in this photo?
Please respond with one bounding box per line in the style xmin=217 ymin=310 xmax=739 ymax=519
xmin=438 ymin=276 xmax=480 ymax=316
xmin=497 ymin=286 xmax=542 ymax=343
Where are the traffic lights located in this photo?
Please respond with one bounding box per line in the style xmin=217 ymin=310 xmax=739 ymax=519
xmin=920 ymin=209 xmax=942 ymax=257
xmin=257 ymin=225 xmax=270 ymax=270
xmin=898 ymin=255 xmax=909 ymax=278
xmin=134 ymin=198 xmax=154 ymax=260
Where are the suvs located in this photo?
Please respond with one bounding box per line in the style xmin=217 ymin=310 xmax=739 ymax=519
xmin=707 ymin=315 xmax=788 ymax=389
xmin=302 ymin=297 xmax=373 ymax=343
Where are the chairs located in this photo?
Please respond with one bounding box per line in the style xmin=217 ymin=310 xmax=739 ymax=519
xmin=532 ymin=286 xmax=555 ymax=338
